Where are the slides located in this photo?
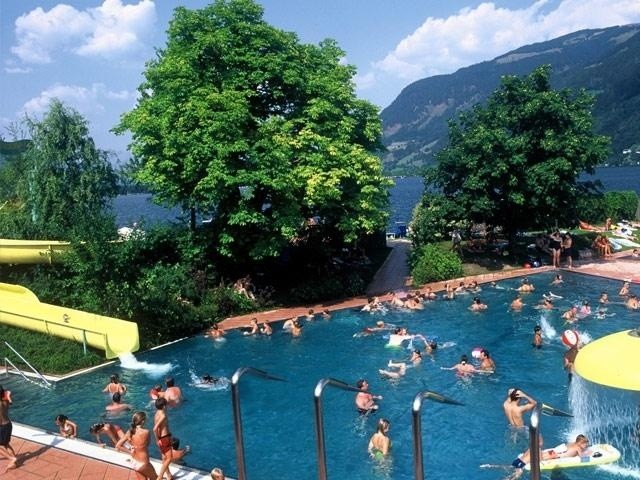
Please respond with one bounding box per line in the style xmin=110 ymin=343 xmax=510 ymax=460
xmin=0 ymin=282 xmax=141 ymax=361
xmin=0 ymin=235 xmax=133 ymax=264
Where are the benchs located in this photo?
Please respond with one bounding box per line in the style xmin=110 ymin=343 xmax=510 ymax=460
xmin=578 ymin=247 xmax=615 ymax=266
